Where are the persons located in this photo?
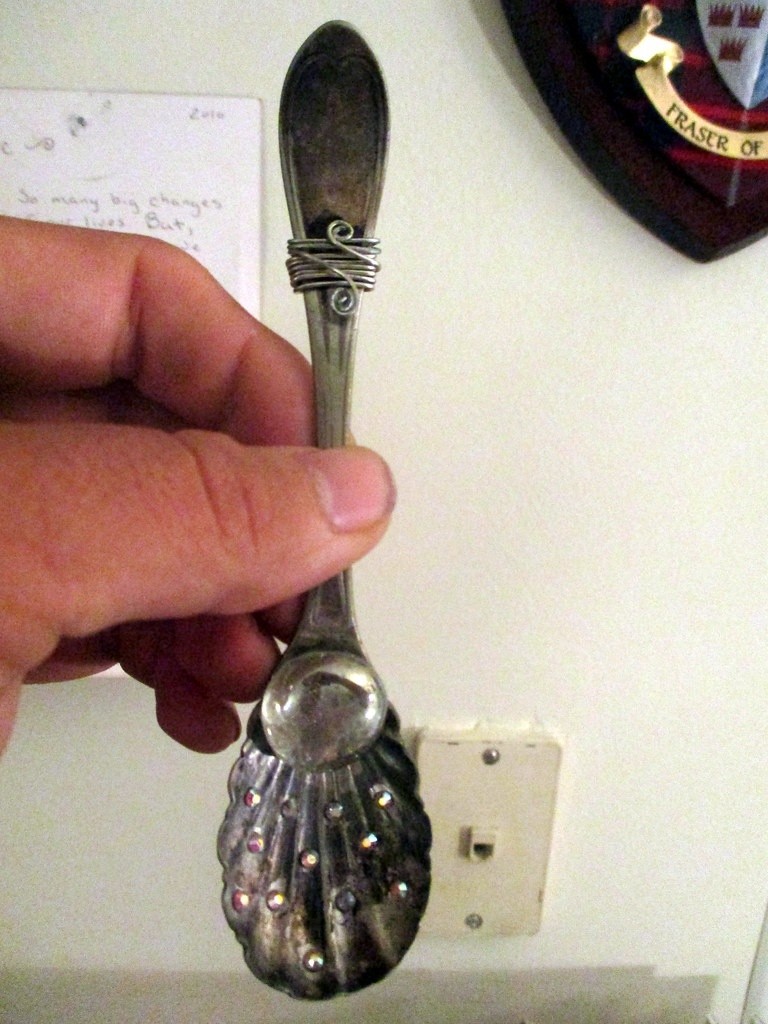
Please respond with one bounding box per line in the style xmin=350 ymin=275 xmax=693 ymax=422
xmin=0 ymin=213 xmax=397 ymax=757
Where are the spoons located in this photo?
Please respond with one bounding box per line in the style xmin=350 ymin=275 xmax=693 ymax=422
xmin=216 ymin=20 xmax=432 ymax=1001
